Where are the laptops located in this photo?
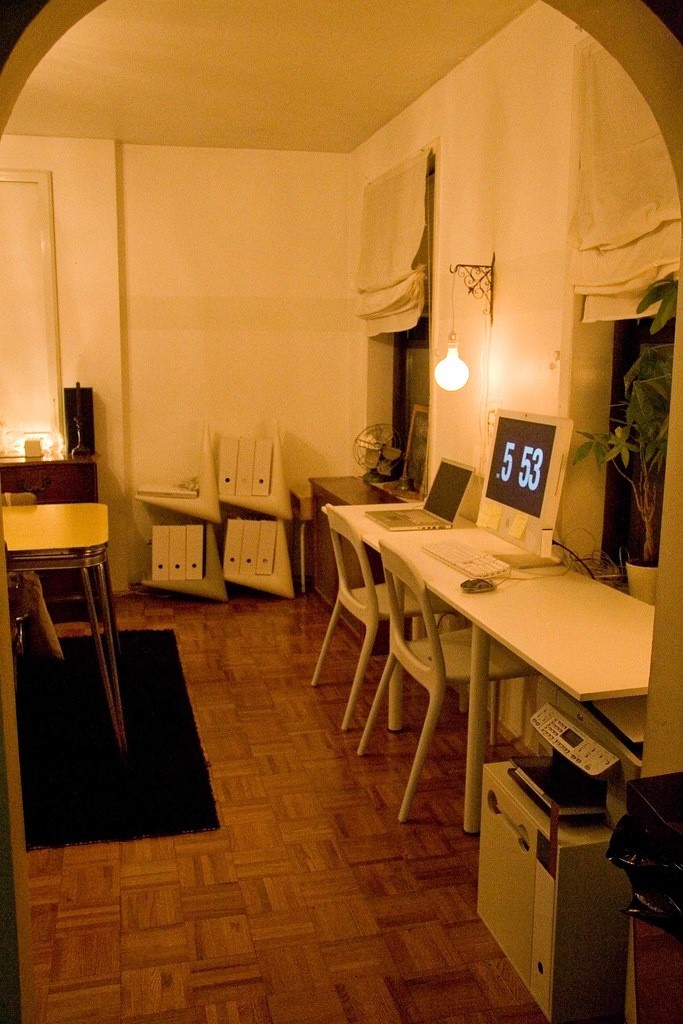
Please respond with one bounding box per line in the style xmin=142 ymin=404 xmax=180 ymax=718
xmin=365 ymin=458 xmax=476 ymax=531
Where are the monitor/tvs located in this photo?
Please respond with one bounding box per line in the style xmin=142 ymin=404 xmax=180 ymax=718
xmin=476 ymin=408 xmax=574 ymax=569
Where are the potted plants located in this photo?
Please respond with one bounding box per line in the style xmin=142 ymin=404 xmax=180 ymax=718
xmin=564 ymin=274 xmax=678 ymax=606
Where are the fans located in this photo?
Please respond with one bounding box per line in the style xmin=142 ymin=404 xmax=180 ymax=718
xmin=352 ymin=424 xmax=405 ymax=483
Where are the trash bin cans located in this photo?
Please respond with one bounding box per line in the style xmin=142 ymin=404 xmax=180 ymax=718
xmin=626 ymin=771 xmax=683 ymax=1024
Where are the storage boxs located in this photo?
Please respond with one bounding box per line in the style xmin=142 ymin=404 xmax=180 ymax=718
xmin=475 ymin=762 xmax=633 ymax=1024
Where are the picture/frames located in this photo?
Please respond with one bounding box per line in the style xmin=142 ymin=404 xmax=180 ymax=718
xmin=402 ymin=403 xmax=430 ymax=494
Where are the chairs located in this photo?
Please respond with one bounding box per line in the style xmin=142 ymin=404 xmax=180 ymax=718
xmin=309 ymin=501 xmax=469 ymax=733
xmin=2 ymin=491 xmax=65 ymax=695
xmin=357 ymin=538 xmax=543 ymax=825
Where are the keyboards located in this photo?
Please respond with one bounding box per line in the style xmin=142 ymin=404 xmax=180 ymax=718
xmin=422 ymin=537 xmax=510 ymax=578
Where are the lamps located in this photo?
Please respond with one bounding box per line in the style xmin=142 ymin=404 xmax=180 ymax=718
xmin=434 ymin=252 xmax=497 ymax=393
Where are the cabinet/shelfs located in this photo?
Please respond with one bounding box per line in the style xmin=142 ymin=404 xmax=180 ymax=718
xmin=134 ymin=418 xmax=297 ymax=603
xmin=0 ymin=447 xmax=106 ymax=623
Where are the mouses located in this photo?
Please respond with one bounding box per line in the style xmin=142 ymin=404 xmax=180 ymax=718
xmin=460 ymin=578 xmax=495 ymax=594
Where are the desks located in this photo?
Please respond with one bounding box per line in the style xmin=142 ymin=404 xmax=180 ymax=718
xmin=3 ymin=504 xmax=131 ymax=758
xmin=286 ymin=476 xmax=655 ymax=840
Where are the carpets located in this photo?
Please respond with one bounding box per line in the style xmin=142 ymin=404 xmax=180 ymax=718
xmin=12 ymin=626 xmax=223 ymax=851
